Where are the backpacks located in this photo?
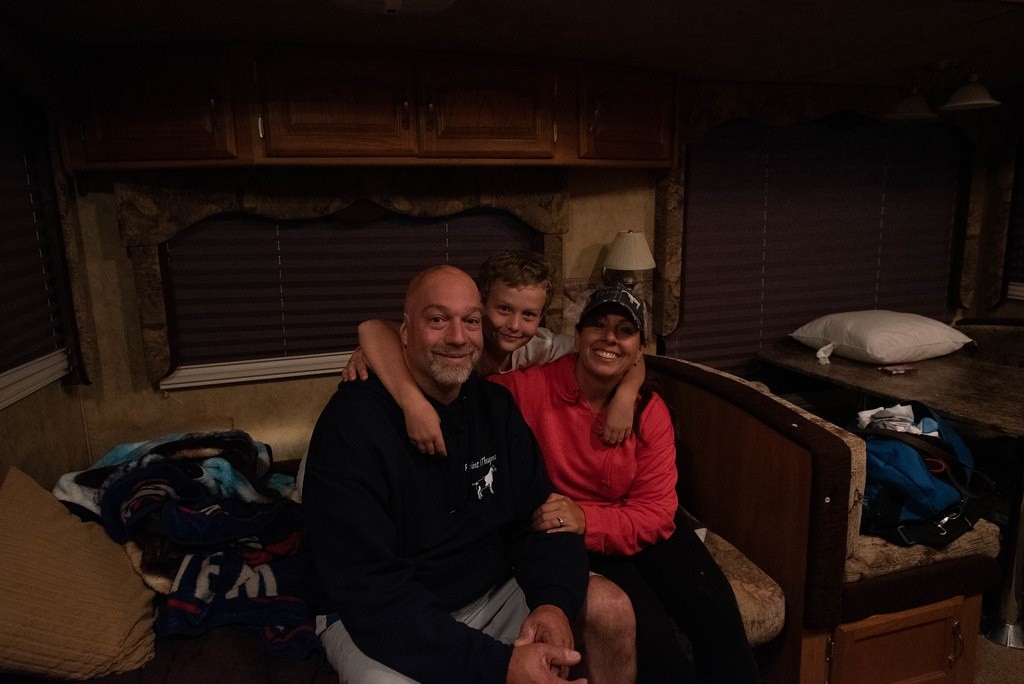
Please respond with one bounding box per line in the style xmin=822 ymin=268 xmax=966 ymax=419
xmin=851 ymin=400 xmax=998 ymax=550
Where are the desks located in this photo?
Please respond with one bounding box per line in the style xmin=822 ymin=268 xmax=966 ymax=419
xmin=765 ymin=338 xmax=1024 ymax=651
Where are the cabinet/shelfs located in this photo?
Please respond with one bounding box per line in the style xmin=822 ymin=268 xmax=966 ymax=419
xmin=41 ymin=55 xmax=686 ymax=172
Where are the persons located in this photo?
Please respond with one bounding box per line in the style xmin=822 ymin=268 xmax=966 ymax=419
xmin=302 ymin=263 xmax=637 ymax=684
xmin=340 ymin=285 xmax=760 ymax=684
xmin=358 ymin=250 xmax=647 ymax=457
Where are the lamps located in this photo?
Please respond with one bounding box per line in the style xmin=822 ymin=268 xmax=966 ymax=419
xmin=937 ymin=65 xmax=1001 ymax=111
xmin=600 ymin=233 xmax=659 ymax=306
xmin=881 ymin=82 xmax=939 ymax=121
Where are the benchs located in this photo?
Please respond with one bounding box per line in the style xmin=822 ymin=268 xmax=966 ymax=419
xmin=639 ymin=352 xmax=1000 ymax=684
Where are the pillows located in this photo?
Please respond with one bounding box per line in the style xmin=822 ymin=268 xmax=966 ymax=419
xmin=0 ymin=471 xmax=160 ymax=679
xmin=787 ymin=310 xmax=970 ymax=364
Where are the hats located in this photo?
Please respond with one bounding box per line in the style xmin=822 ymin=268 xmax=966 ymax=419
xmin=580 ymin=284 xmax=647 ymax=342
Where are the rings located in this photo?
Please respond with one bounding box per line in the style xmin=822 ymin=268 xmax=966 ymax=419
xmin=557 ymin=517 xmax=563 ymax=526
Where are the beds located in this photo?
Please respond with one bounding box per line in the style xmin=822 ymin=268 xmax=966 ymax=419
xmin=0 ymin=352 xmax=868 ymax=684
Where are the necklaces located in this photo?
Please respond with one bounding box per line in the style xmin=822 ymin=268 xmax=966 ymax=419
xmin=580 ymin=385 xmax=597 ymax=424
